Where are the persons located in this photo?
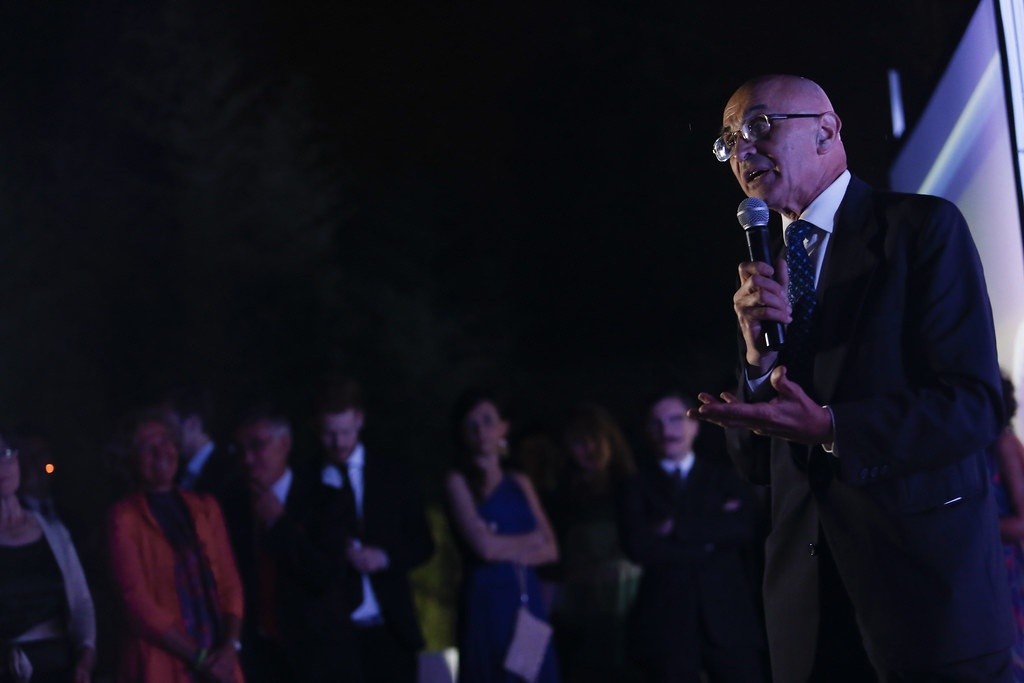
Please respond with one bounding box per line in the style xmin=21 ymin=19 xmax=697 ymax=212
xmin=0 ymin=376 xmax=1024 ymax=683
xmin=687 ymin=75 xmax=1024 ymax=683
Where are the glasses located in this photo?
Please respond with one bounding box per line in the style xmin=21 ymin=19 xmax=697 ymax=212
xmin=712 ymin=113 xmax=821 ymax=162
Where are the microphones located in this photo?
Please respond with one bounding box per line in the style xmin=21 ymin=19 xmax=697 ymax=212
xmin=737 ymin=196 xmax=784 ymax=351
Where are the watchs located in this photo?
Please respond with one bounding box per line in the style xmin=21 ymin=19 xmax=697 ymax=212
xmin=223 ymin=638 xmax=243 ymax=653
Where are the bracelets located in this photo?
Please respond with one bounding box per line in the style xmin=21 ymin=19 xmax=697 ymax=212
xmin=194 ymin=648 xmax=208 ymax=670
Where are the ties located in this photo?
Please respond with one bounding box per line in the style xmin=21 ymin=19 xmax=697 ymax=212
xmin=335 ymin=463 xmax=364 ymax=613
xmin=785 ymin=219 xmax=816 ymax=403
xmin=671 ymin=466 xmax=682 ymax=481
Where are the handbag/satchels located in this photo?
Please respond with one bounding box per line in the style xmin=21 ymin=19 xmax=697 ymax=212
xmin=502 ymin=562 xmax=553 ymax=683
